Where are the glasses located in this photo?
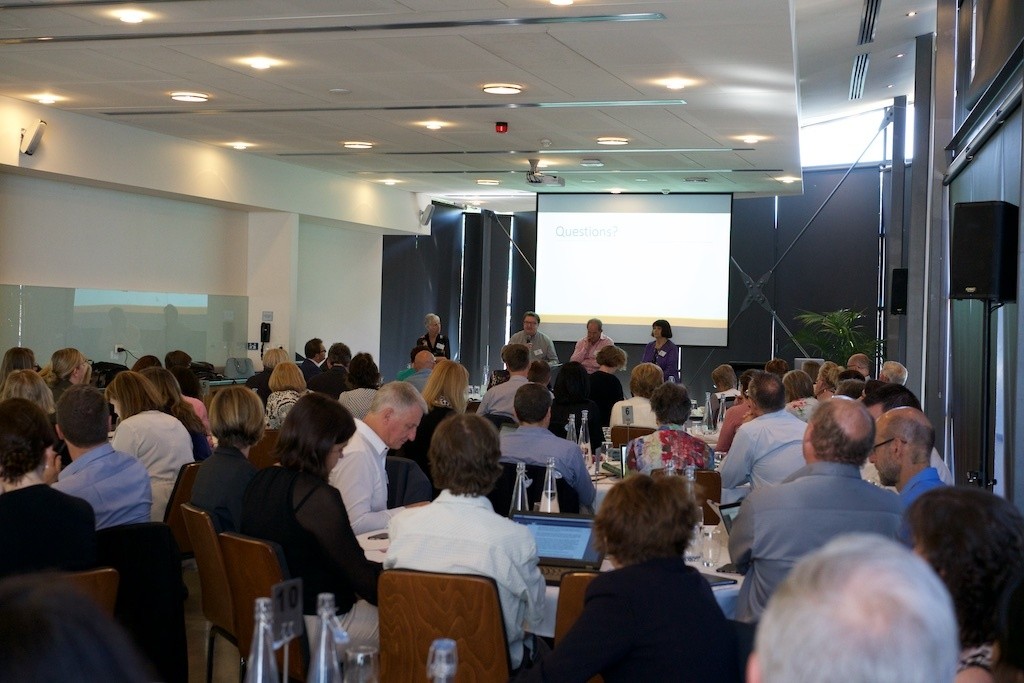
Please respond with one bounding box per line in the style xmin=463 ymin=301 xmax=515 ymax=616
xmin=77 ymin=360 xmax=88 ymax=370
xmin=524 ymin=320 xmax=537 ymax=325
xmin=321 ymin=349 xmax=326 ymax=353
xmin=34 ymin=363 xmax=39 ymax=368
xmin=713 ymin=384 xmax=717 ymax=389
xmin=745 ymin=389 xmax=757 ymax=405
xmin=867 ymin=437 xmax=908 ymax=456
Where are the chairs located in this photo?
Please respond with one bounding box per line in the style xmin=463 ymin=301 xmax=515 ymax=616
xmin=60 ymin=430 xmax=722 ymax=683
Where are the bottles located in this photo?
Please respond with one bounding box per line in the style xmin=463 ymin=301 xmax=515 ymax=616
xmin=539 ymin=456 xmax=560 ymax=513
xmin=665 ymin=459 xmax=675 ymax=476
xmin=567 ymin=414 xmax=577 ymax=444
xmin=307 ymin=593 xmax=342 ymax=683
xmin=702 ymin=392 xmax=714 ymax=432
xmin=245 ymin=597 xmax=279 ymax=683
xmin=578 ymin=410 xmax=592 ymax=470
xmin=684 ymin=466 xmax=695 ymax=495
xmin=509 ymin=461 xmax=529 ymax=515
xmin=483 ymin=374 xmax=487 ymax=385
xmin=429 ymin=638 xmax=458 ymax=683
xmin=716 ymin=394 xmax=726 ymax=433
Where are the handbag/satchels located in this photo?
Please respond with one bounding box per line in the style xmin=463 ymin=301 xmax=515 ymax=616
xmin=224 ymin=357 xmax=255 ymax=379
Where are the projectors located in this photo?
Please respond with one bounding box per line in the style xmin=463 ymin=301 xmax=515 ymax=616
xmin=527 ymin=175 xmax=566 ymax=187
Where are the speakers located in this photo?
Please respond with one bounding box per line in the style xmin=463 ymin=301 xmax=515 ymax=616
xmin=421 ymin=204 xmax=436 ymax=225
xmin=21 ymin=119 xmax=47 ymax=155
xmin=890 ymin=267 xmax=908 ymax=315
xmin=949 ymin=201 xmax=1020 ymax=304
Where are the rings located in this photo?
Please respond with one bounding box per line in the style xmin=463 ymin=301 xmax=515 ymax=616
xmin=45 ymin=465 xmax=49 ymax=469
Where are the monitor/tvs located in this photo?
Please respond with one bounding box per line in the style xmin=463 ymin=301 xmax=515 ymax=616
xmin=504 ymin=509 xmax=621 ymax=567
xmin=719 ymin=502 xmax=741 ymax=533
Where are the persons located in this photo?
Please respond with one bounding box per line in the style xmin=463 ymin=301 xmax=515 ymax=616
xmin=727 ymin=395 xmax=904 ymax=625
xmin=499 ymin=383 xmax=596 ymax=506
xmin=383 ymin=413 xmax=547 ymax=676
xmin=50 ymin=384 xmax=154 ymax=531
xmin=901 ymin=487 xmax=1024 ymax=683
xmin=0 ymin=338 xmax=951 ymax=683
xmin=417 ymin=313 xmax=450 ymax=362
xmin=235 ymin=392 xmax=385 ymax=653
xmin=0 ymin=396 xmax=97 ymax=580
xmin=526 ymin=473 xmax=740 ymax=683
xmin=327 ymin=381 xmax=432 ymax=536
xmin=641 ymin=320 xmax=680 ymax=384
xmin=570 ymin=319 xmax=614 ymax=374
xmin=509 ymin=311 xmax=560 ymax=367
xmin=869 ymin=406 xmax=948 ymax=507
xmin=744 ymin=530 xmax=959 ymax=683
xmin=163 ymin=385 xmax=264 ymax=535
xmin=626 ymin=382 xmax=714 ymax=480
xmin=718 ymin=373 xmax=808 ymax=491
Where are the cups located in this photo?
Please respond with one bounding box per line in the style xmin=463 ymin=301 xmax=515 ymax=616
xmin=474 ymin=386 xmax=479 ymax=393
xmin=691 ymin=400 xmax=697 ymax=409
xmin=700 ymin=525 xmax=721 ymax=567
xmin=601 ymin=442 xmax=613 ymax=463
xmin=698 ymin=506 xmax=704 ymax=530
xmin=714 ymin=452 xmax=727 ymax=463
xmin=343 ymin=646 xmax=379 ymax=683
xmin=602 ymin=427 xmax=611 ymax=442
xmin=682 ymin=422 xmax=687 ymax=432
xmin=691 ymin=421 xmax=702 ymax=434
xmin=683 ymin=523 xmax=703 ymax=561
xmin=534 ymin=502 xmax=540 ymax=511
xmin=469 ymin=386 xmax=473 ymax=393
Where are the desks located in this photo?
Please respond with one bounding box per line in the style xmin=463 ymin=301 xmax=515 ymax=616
xmin=551 ymin=461 xmax=733 ymax=569
xmin=354 ymin=515 xmax=746 ymax=638
